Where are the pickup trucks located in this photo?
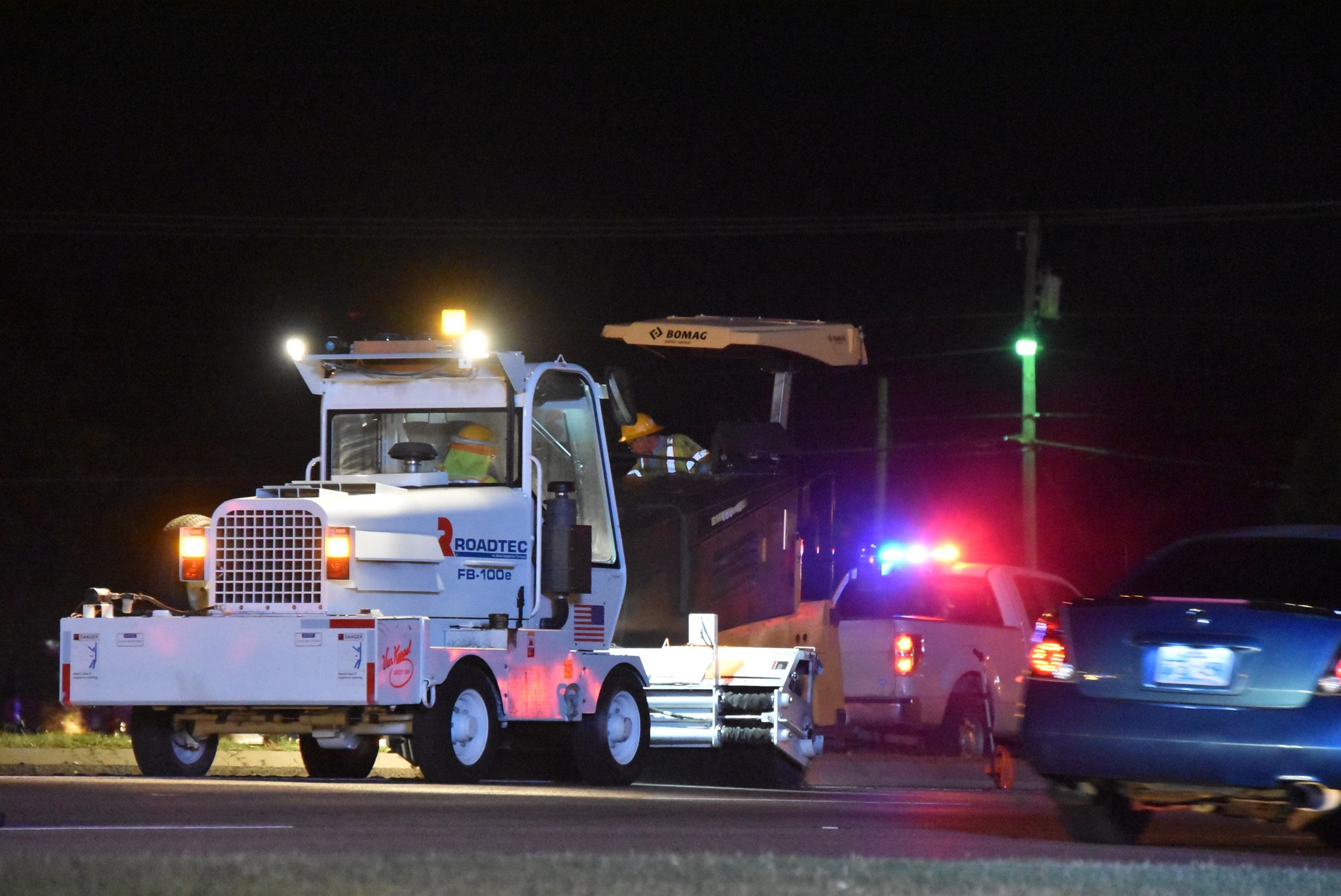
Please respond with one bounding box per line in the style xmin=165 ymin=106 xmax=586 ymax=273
xmin=833 ymin=562 xmax=1085 ymax=756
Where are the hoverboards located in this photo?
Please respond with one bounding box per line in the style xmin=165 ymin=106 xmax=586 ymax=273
xmin=960 ymin=649 xmax=1014 ymax=792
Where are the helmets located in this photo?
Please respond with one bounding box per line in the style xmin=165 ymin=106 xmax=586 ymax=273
xmin=618 ymin=413 xmax=662 ymax=442
xmin=449 ymin=424 xmax=500 ymax=456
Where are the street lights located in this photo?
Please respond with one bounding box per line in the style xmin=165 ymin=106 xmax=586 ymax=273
xmin=1015 ymin=331 xmax=1038 ymax=568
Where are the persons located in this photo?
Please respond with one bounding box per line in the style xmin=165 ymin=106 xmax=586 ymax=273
xmin=619 ymin=412 xmax=710 ymax=481
xmin=434 ymin=423 xmax=499 ymax=484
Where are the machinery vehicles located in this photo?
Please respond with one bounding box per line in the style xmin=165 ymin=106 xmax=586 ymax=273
xmin=58 ymin=299 xmax=867 ymax=788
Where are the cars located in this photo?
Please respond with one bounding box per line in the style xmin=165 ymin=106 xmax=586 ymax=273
xmin=1019 ymin=521 xmax=1341 ymax=835
